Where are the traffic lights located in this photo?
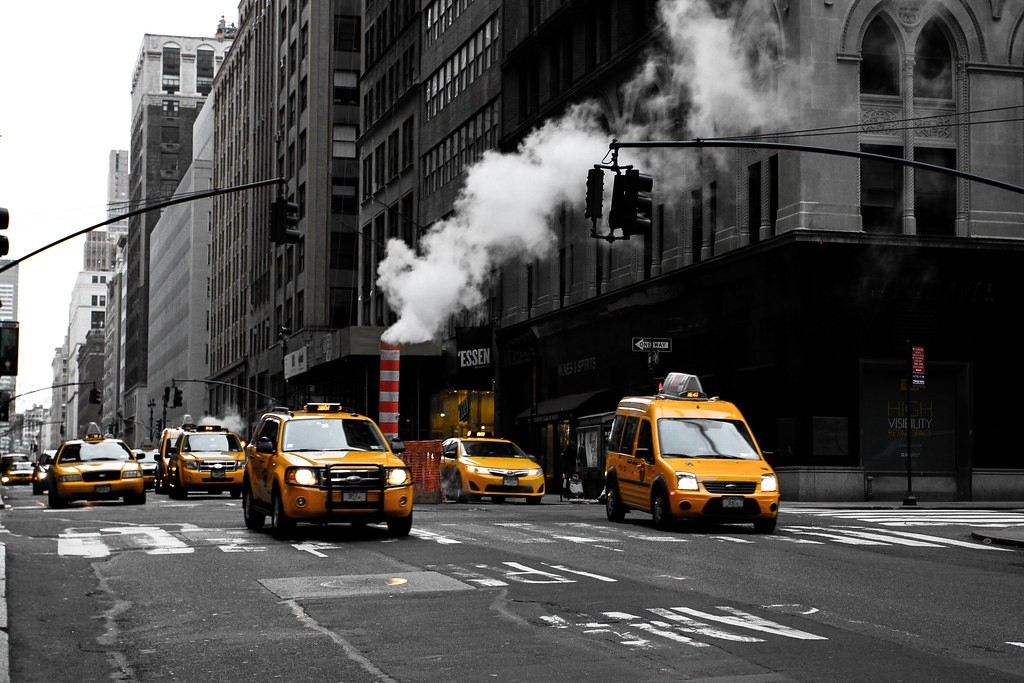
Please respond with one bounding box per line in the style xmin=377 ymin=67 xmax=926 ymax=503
xmin=94 ymin=389 xmax=101 ymax=405
xmin=624 ymin=169 xmax=653 ymax=237
xmin=0 ymin=207 xmax=10 ymax=257
xmin=34 ymin=444 xmax=39 ymax=452
xmin=174 ymin=386 xmax=183 ymax=407
xmin=60 ymin=425 xmax=65 ymax=435
xmin=276 ymin=200 xmax=301 ymax=247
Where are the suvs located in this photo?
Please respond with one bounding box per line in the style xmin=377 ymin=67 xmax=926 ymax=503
xmin=241 ymin=403 xmax=414 ymax=540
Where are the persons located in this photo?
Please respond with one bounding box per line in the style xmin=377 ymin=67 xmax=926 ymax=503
xmin=560 ymin=441 xmax=577 ymax=502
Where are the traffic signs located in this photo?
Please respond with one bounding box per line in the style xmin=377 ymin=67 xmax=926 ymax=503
xmin=631 ymin=337 xmax=673 ymax=353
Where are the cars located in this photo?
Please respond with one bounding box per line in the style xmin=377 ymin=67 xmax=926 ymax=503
xmin=167 ymin=425 xmax=246 ymax=501
xmin=131 ymin=448 xmax=160 ymax=484
xmin=427 ymin=431 xmax=546 ymax=506
xmin=47 ymin=433 xmax=146 ymax=509
xmin=153 ymin=424 xmax=198 ymax=495
xmin=31 ymin=450 xmax=57 ymax=495
xmin=2 ymin=453 xmax=34 ymax=486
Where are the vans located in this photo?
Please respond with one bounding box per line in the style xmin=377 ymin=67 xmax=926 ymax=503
xmin=604 ymin=372 xmax=780 ymax=532
